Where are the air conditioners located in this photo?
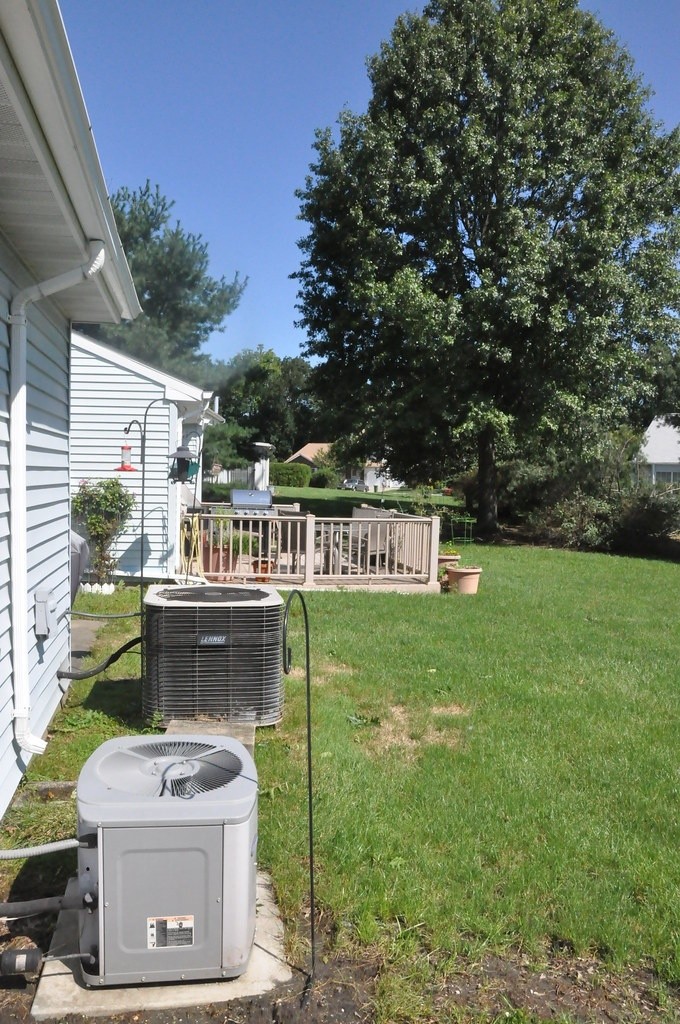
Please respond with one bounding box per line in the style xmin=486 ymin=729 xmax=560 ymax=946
xmin=139 ymin=583 xmax=288 ymax=731
xmin=75 ymin=730 xmax=262 ymax=990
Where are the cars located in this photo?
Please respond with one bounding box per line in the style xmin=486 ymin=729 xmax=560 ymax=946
xmin=337 ymin=479 xmax=368 ymax=493
xmin=167 ymin=431 xmax=200 ymax=583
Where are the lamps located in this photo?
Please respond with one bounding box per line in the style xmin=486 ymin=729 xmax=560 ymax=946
xmin=166 ymin=445 xmax=200 ymax=486
xmin=114 ymin=446 xmax=138 ymax=472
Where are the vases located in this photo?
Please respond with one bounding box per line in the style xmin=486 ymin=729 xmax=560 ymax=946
xmin=446 ymin=564 xmax=483 ymax=595
xmin=252 ymin=559 xmax=274 ymax=582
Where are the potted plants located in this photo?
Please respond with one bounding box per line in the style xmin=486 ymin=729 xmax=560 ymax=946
xmin=437 ymin=542 xmax=462 ymax=580
xmin=197 ymin=506 xmax=260 ymax=582
